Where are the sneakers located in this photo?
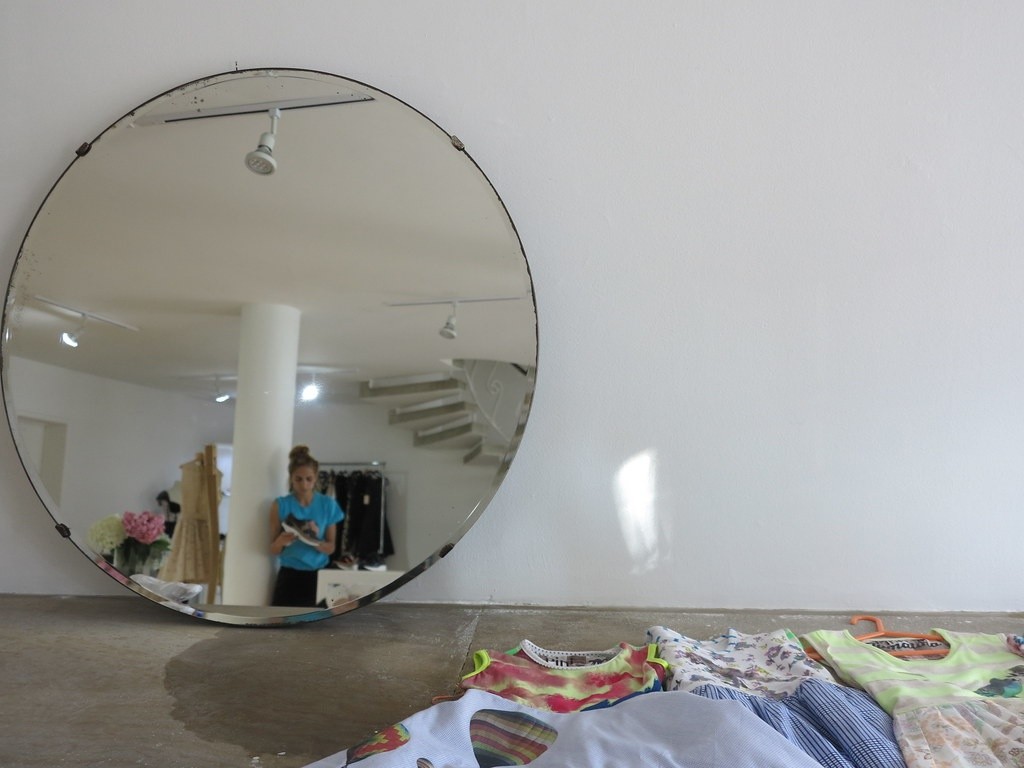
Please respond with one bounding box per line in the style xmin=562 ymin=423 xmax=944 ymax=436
xmin=281 ymin=512 xmax=321 ymax=547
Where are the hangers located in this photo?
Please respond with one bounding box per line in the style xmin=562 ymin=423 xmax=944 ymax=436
xmin=805 ymin=615 xmax=950 ymax=662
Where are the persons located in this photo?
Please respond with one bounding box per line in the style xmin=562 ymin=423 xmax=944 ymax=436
xmin=270 ymin=446 xmax=345 ymax=607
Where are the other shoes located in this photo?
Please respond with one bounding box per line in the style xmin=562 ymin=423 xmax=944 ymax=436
xmin=327 ymin=553 xmax=359 ymax=571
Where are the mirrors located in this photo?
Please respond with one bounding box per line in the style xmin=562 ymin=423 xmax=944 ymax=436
xmin=0 ymin=67 xmax=538 ymax=630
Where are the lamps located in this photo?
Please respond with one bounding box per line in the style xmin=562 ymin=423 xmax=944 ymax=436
xmin=439 ymin=303 xmax=457 ymax=339
xmin=63 ymin=313 xmax=88 ymax=347
xmin=243 ymin=108 xmax=285 ymax=174
